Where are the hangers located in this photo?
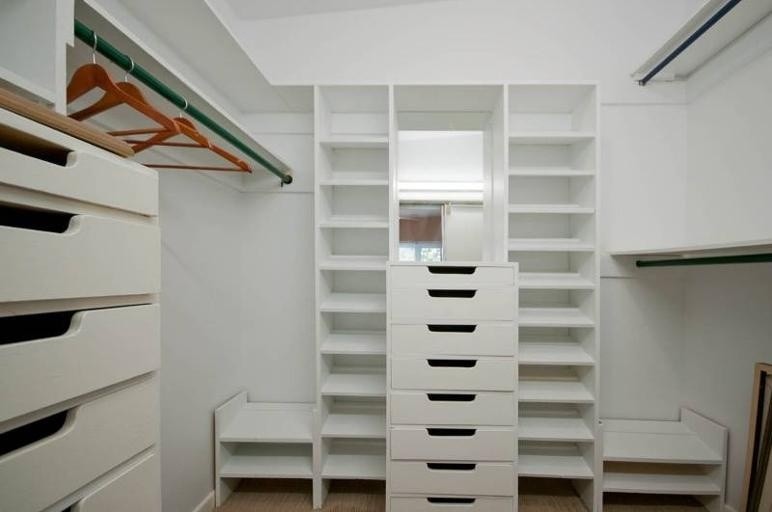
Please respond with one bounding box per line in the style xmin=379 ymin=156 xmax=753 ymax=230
xmin=105 ymin=51 xmax=213 ymax=147
xmin=66 ymin=29 xmax=179 ymax=145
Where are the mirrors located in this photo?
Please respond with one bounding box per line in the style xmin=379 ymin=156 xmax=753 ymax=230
xmin=391 ymin=84 xmax=505 ymax=263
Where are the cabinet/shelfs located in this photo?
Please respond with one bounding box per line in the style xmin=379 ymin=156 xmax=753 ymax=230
xmin=384 ymin=261 xmax=520 ymax=512
xmin=211 ymin=390 xmax=318 ymax=510
xmin=0 ymin=107 xmax=160 ymax=511
xmin=506 ymin=84 xmax=601 ymax=511
xmin=599 ymin=405 xmax=733 ymax=511
xmin=133 ymin=97 xmax=253 ymax=174
xmin=314 ymin=85 xmax=390 ymax=512
